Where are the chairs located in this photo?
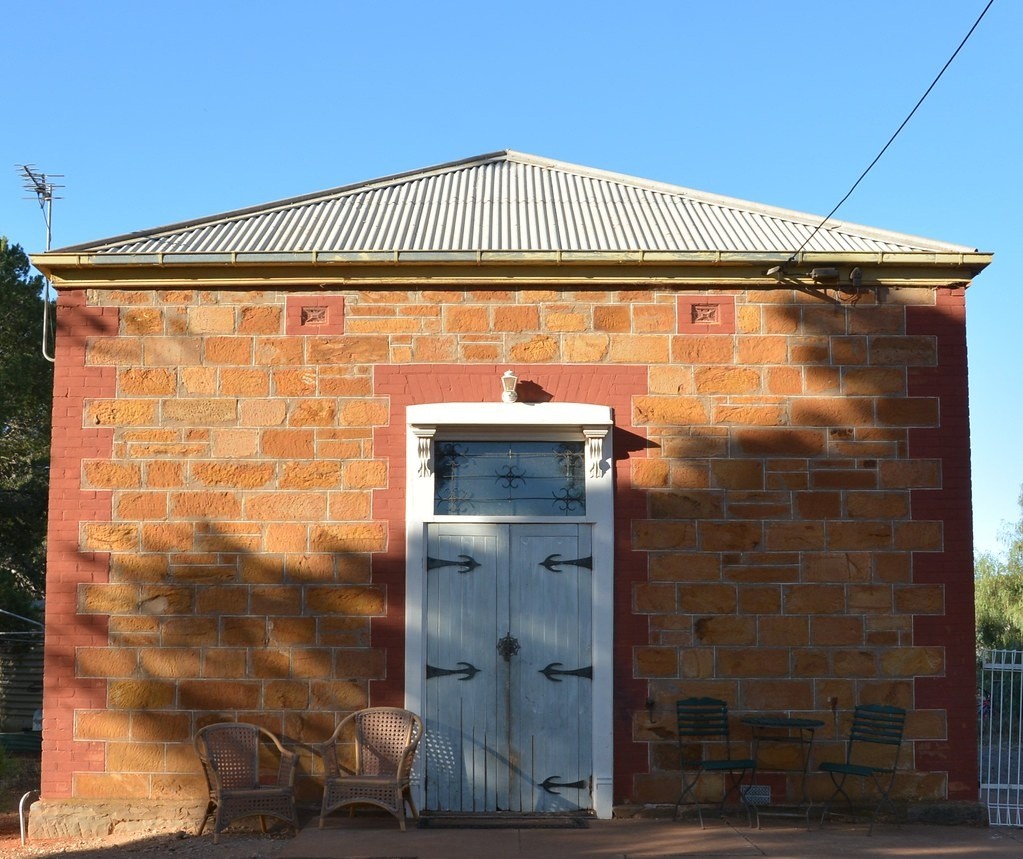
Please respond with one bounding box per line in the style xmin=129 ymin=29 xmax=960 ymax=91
xmin=671 ymin=696 xmax=760 ymax=831
xmin=316 ymin=707 xmax=422 ymax=832
xmin=192 ymin=722 xmax=299 ymax=846
xmin=817 ymin=704 xmax=906 ymax=835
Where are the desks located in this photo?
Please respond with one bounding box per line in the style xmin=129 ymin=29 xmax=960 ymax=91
xmin=741 ymin=717 xmax=824 ymax=832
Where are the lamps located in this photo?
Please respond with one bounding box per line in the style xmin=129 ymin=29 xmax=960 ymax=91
xmin=501 ymin=369 xmax=518 ymax=403
